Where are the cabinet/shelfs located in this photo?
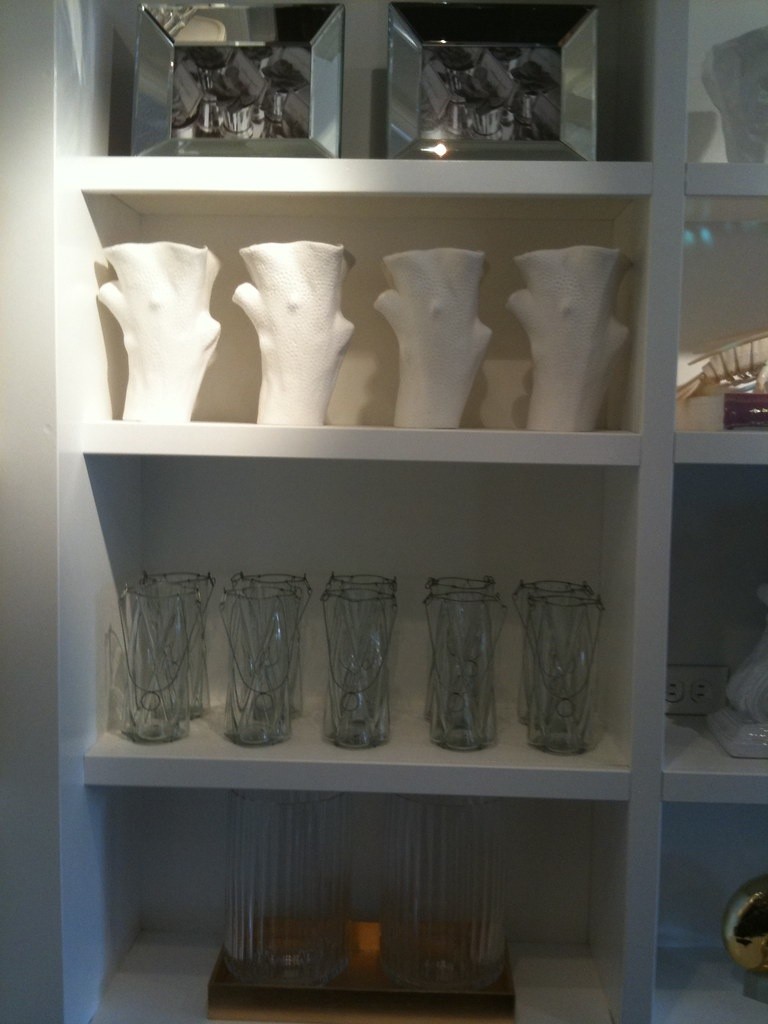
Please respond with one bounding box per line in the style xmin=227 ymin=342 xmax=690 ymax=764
xmin=1 ymin=0 xmax=767 ymax=1022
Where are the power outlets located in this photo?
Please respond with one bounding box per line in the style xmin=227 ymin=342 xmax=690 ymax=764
xmin=666 ymin=664 xmax=728 ymax=715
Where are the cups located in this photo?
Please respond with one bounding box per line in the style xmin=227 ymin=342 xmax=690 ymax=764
xmin=512 ymin=578 xmax=595 ymax=724
xmin=231 ymin=570 xmax=312 ymax=721
xmin=320 ymin=588 xmax=392 ymax=749
xmin=524 ymin=594 xmax=601 ymax=754
xmin=423 ymin=592 xmax=506 ymax=751
xmin=424 ymin=574 xmax=497 ymax=723
xmin=222 ymin=790 xmax=350 ymax=987
xmin=388 ymin=793 xmax=512 ymax=992
xmin=217 ymin=588 xmax=303 ymax=746
xmin=134 ymin=570 xmax=216 ymax=716
xmin=701 ymin=24 xmax=768 ymax=163
xmin=323 ymin=569 xmax=398 ymax=742
xmin=117 ymin=581 xmax=199 ymax=744
